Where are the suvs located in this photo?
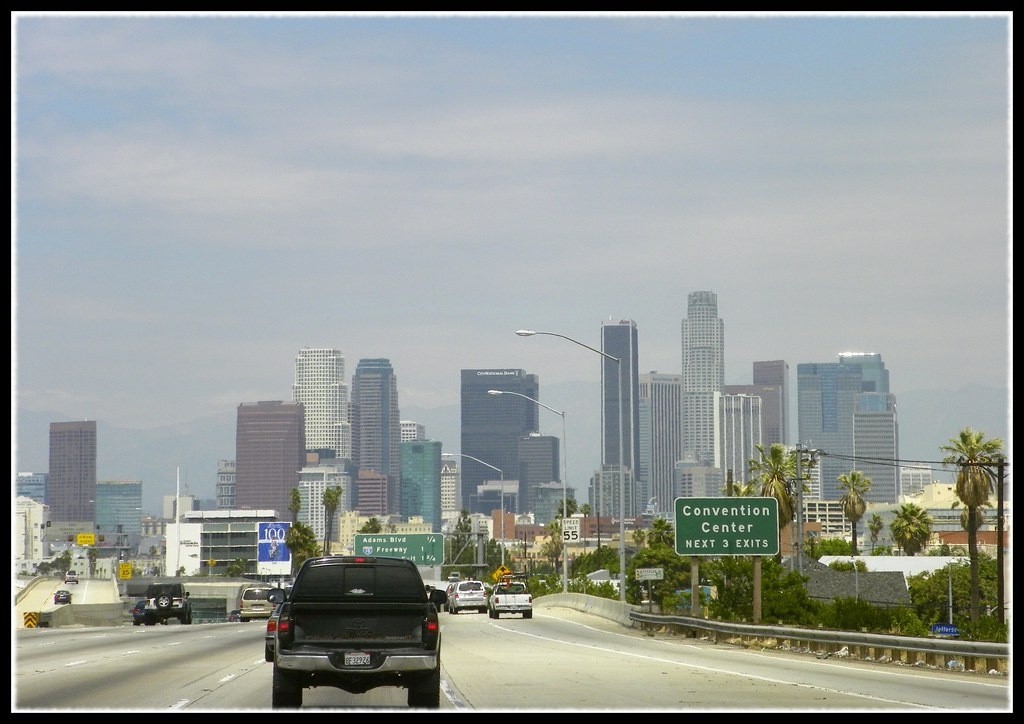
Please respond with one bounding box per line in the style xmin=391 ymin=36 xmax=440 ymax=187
xmin=145 ymin=583 xmax=193 ymax=625
xmin=241 ymin=585 xmax=287 ymax=622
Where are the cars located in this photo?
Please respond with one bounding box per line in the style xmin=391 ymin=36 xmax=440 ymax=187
xmin=53 ymin=590 xmax=73 ymax=603
xmin=133 ymin=602 xmax=167 ymax=626
xmin=227 ymin=610 xmax=240 ymax=621
xmin=64 ymin=570 xmax=80 ymax=584
xmin=425 ymin=584 xmax=436 ymax=599
xmin=444 ymin=583 xmax=454 ymax=611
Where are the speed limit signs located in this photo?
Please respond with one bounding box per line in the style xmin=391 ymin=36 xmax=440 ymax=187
xmin=562 ymin=518 xmax=580 ymax=545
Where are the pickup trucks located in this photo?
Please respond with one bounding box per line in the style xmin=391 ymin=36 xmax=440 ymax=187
xmin=488 ymin=581 xmax=534 ymax=619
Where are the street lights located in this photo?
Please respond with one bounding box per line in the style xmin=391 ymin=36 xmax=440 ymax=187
xmin=516 ymin=329 xmax=628 ymax=602
xmin=489 ymin=390 xmax=570 ymax=593
xmin=441 ymin=453 xmax=505 ymax=570
xmin=89 ymin=501 xmax=120 ymax=558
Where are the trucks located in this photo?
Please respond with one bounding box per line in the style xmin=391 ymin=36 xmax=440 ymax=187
xmin=449 ymin=581 xmax=488 ymax=614
xmin=267 ymin=555 xmax=448 ymax=709
